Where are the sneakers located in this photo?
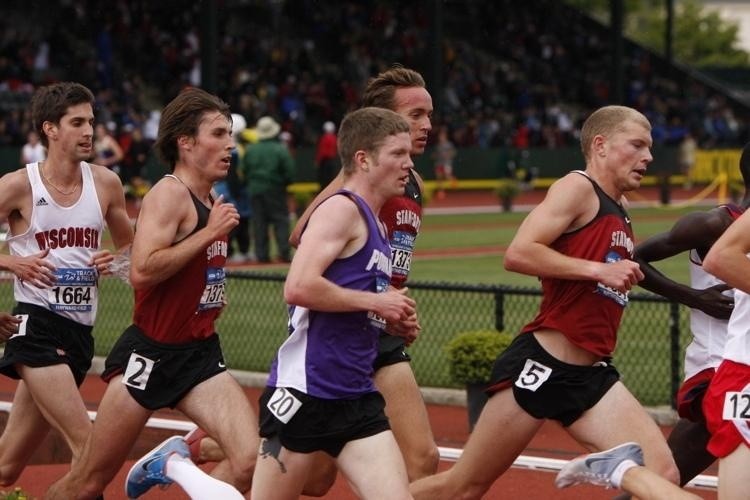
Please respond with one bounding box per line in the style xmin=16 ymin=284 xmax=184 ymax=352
xmin=124 ymin=428 xmax=209 ymax=499
xmin=555 ymin=442 xmax=644 ymax=489
xmin=0 ymin=487 xmax=27 ymax=500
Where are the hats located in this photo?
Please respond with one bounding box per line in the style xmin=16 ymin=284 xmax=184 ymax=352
xmin=256 ymin=115 xmax=281 ymax=140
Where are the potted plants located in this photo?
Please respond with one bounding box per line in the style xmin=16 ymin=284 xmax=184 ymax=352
xmin=495 ymin=183 xmax=519 ymax=213
xmin=442 ymin=327 xmax=514 ymax=434
xmin=294 ymin=192 xmax=310 ymax=217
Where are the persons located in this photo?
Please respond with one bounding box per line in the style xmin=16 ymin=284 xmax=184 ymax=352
xmin=0 ymin=0 xmax=750 ymax=499
